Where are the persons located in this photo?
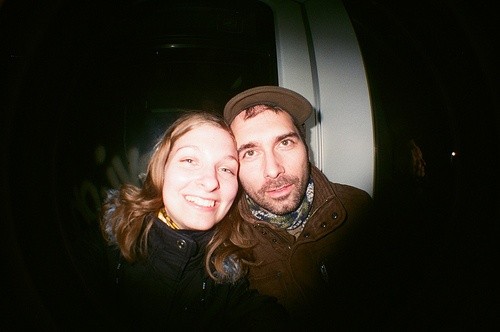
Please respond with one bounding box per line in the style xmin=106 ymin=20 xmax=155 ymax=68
xmin=224 ymin=86 xmax=419 ymax=332
xmin=33 ymin=113 xmax=240 ymax=332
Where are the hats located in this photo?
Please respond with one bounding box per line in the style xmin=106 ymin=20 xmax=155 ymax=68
xmin=225 ymin=86 xmax=314 ymax=138
